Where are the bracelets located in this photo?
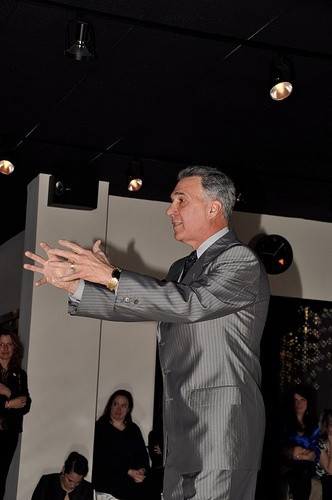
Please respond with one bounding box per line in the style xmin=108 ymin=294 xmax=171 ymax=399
xmin=8 ymin=401 xmax=10 ymax=409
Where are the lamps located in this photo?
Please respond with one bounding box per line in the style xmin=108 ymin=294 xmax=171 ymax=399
xmin=0 ymin=22 xmax=295 ymax=199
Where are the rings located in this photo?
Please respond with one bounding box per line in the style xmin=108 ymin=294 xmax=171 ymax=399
xmin=71 ymin=262 xmax=74 ymax=269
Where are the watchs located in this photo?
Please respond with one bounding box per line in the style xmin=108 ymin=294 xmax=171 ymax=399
xmin=106 ymin=265 xmax=123 ymax=292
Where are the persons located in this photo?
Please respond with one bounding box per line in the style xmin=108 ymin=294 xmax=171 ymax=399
xmin=0 ymin=330 xmax=33 ymax=499
xmin=93 ymin=389 xmax=150 ymax=500
xmin=286 ymin=385 xmax=332 ymax=500
xmin=23 ymin=168 xmax=269 ymax=500
xmin=147 ymin=410 xmax=164 ymax=500
xmin=31 ymin=452 xmax=94 ymax=500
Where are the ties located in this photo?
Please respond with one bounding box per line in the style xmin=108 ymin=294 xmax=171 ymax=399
xmin=179 ymin=250 xmax=198 ymax=284
